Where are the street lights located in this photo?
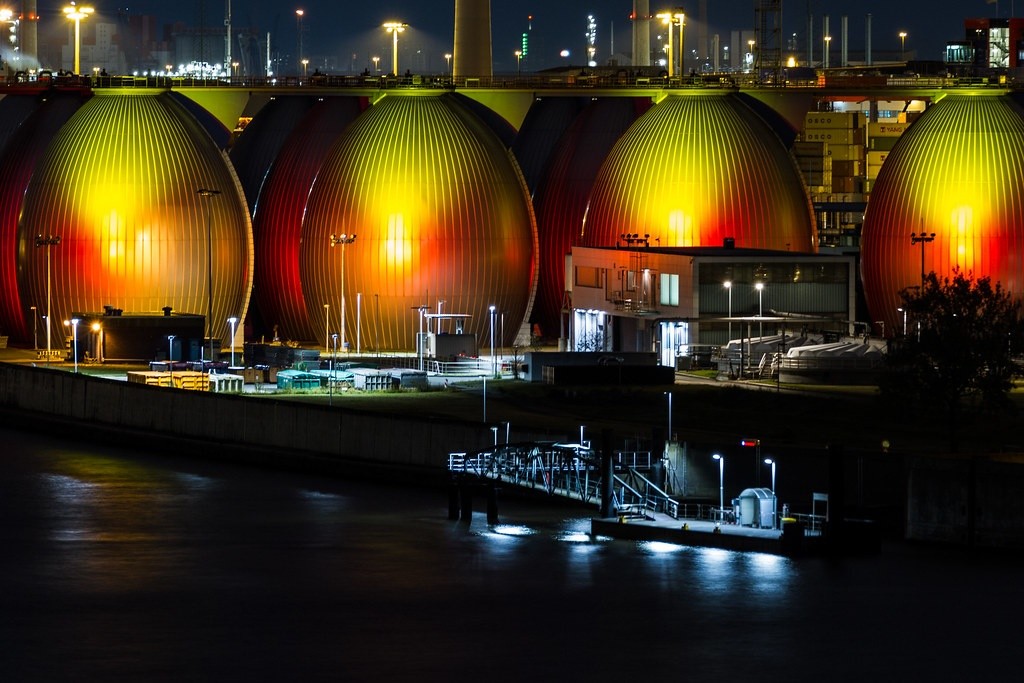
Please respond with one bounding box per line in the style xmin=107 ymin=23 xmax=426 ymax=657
xmin=31 ymin=306 xmax=38 ymax=352
xmin=35 ymin=234 xmax=61 ymax=353
xmin=663 ymin=391 xmax=672 ymax=441
xmin=64 ymin=319 xmax=78 ymax=374
xmin=444 ymin=53 xmax=452 ymax=72
xmin=372 ymin=56 xmax=380 ymax=74
xmin=302 ymin=59 xmax=310 ymax=78
xmin=910 ymin=232 xmax=934 ymax=297
xmin=514 ymin=51 xmax=523 ymax=75
xmin=754 ymin=280 xmax=764 ymax=338
xmin=168 ymin=335 xmax=175 ymax=371
xmin=490 ymin=427 xmax=498 ymax=474
xmin=418 ymin=306 xmax=425 ymax=370
xmin=60 ymin=7 xmax=94 ymax=75
xmin=489 ymin=305 xmax=497 ymax=355
xmin=501 ymin=420 xmax=510 ymax=446
xmin=899 ymin=31 xmax=907 ymax=59
xmin=437 ymin=300 xmax=445 ymax=337
xmin=356 ymin=292 xmax=362 ymax=354
xmin=723 ymin=279 xmax=732 ymax=341
xmin=764 ymin=459 xmax=777 ymax=529
xmin=824 ymin=37 xmax=832 ymax=68
xmin=228 ymin=318 xmax=239 ymax=368
xmin=748 ymin=40 xmax=756 ymax=65
xmin=323 ymin=304 xmax=330 ymax=354
xmin=90 ymin=321 xmax=102 ymax=363
xmin=713 ymin=454 xmax=724 ymax=526
xmin=331 ymin=233 xmax=355 ymax=351
xmin=197 ymin=188 xmax=222 ymax=362
xmin=896 ymin=308 xmax=907 ymax=335
xmin=231 ymin=62 xmax=239 ymax=75
